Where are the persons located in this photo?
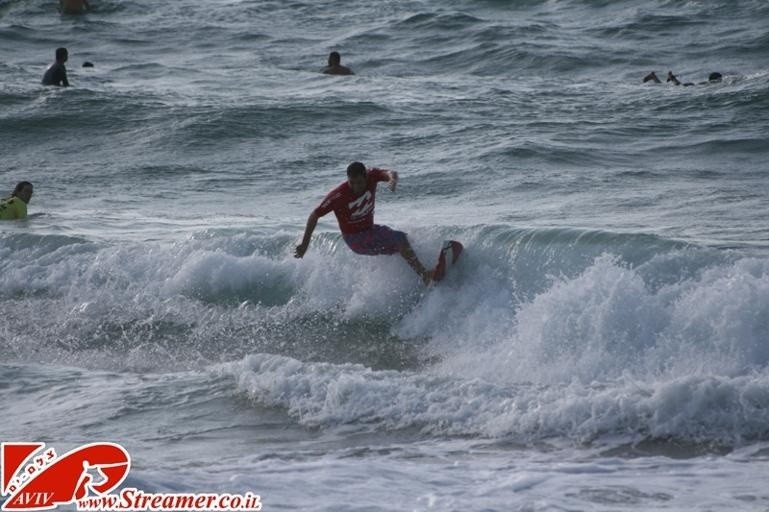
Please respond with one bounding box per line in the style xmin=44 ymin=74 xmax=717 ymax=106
xmin=642 ymin=70 xmax=722 ymax=86
xmin=318 ymin=51 xmax=353 ymax=75
xmin=1 ymin=181 xmax=34 ymax=221
xmin=61 ymin=1 xmax=90 ymax=13
xmin=41 ymin=47 xmax=69 ymax=87
xmin=293 ymin=160 xmax=437 ymax=286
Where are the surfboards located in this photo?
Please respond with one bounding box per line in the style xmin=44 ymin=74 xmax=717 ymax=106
xmin=424 ymin=239 xmax=463 ymax=288
